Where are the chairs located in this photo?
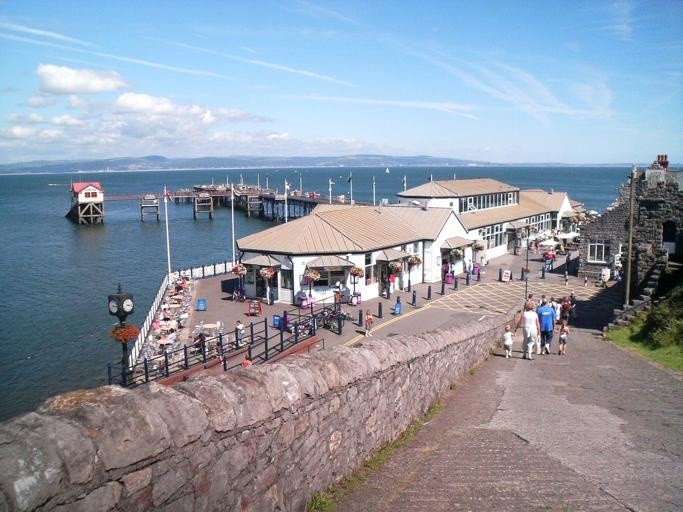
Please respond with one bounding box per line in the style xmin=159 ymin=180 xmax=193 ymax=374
xmin=131 ymin=276 xmax=236 ymax=378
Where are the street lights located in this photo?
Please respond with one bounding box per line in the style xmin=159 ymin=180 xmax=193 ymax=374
xmin=334 ymin=280 xmax=341 ymax=316
xmin=523 ymin=272 xmax=528 ymax=300
xmin=624 ymin=163 xmax=638 ymax=305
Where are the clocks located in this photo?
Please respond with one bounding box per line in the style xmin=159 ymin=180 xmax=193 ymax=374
xmin=106 ymin=282 xmax=140 ymax=389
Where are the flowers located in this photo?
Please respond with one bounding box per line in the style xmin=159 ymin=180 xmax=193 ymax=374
xmin=471 ymin=242 xmax=485 ymax=252
xmin=406 ymin=256 xmax=423 ymax=270
xmin=230 ymin=260 xmax=404 ymax=284
xmin=449 ymin=247 xmax=464 ymax=259
xmin=108 ymin=323 xmax=140 ymax=344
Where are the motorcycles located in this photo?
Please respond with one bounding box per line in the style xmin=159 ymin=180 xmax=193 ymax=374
xmin=294 ymin=307 xmax=345 ymax=337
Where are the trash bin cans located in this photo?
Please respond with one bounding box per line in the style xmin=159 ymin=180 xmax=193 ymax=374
xmin=474 ymin=264 xmax=478 ymax=275
xmin=272 ymin=315 xmax=280 ymax=328
xmin=446 ymin=274 xmax=452 ymax=284
xmin=515 ymin=246 xmax=521 ymax=255
xmin=350 ymin=292 xmax=361 ymax=305
xmin=301 ymin=297 xmax=307 ymax=308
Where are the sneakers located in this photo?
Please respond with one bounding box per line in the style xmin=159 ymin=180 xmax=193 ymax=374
xmin=365 ymin=334 xmax=372 ymax=337
xmin=506 ymin=347 xmax=565 ymax=359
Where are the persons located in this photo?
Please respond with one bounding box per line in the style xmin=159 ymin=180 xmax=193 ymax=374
xmin=468 ymin=259 xmax=473 ymax=282
xmin=514 ymin=292 xmax=578 ymax=360
xmin=136 ymin=271 xmax=192 ymax=372
xmin=236 ymin=321 xmax=245 ymax=344
xmin=193 ymin=333 xmax=208 ymax=353
xmin=479 ymin=257 xmax=485 ymax=274
xmin=566 ymin=253 xmax=571 ymax=268
xmin=502 ymin=325 xmax=515 ymax=358
xmin=559 ymin=243 xmax=564 ymax=254
xmin=365 ymin=310 xmax=373 ymax=337
